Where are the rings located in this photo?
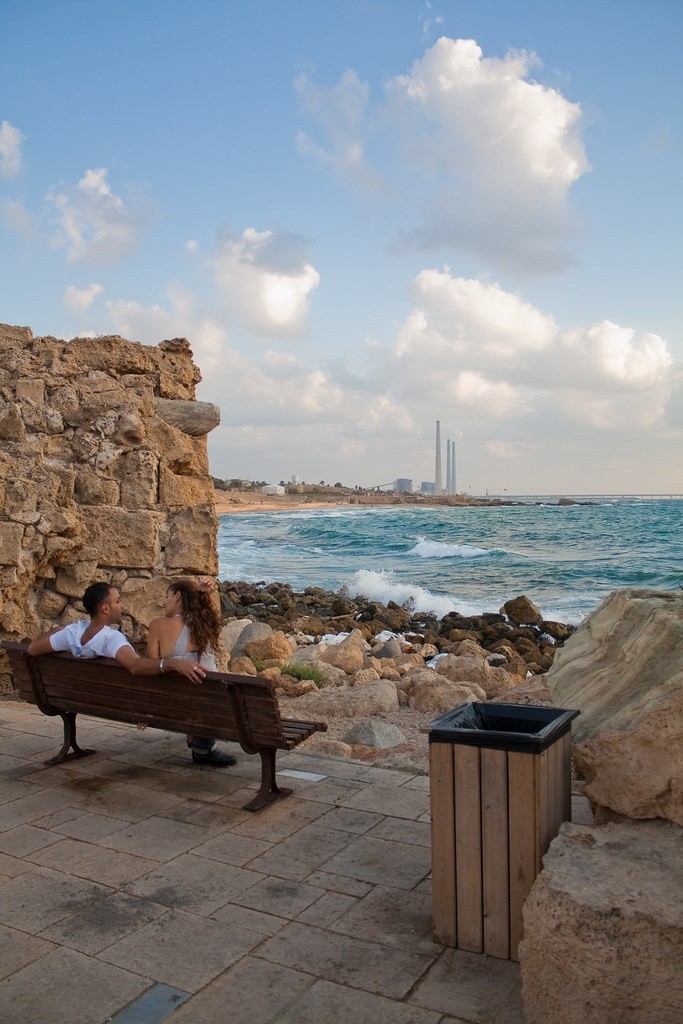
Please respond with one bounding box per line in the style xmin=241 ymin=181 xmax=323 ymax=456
xmin=193 ymin=666 xmax=198 ymax=671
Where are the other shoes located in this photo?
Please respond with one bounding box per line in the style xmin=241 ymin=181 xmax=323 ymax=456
xmin=191 ymin=749 xmax=237 ymax=769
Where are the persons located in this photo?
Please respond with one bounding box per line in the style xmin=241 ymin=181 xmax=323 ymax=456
xmin=28 ymin=582 xmax=209 ymax=685
xmin=137 ymin=579 xmax=238 ymax=767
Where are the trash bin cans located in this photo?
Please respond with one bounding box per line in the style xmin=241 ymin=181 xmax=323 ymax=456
xmin=417 ymin=698 xmax=582 ymax=964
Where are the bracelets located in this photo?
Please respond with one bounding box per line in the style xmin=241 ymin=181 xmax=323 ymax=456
xmin=159 ymin=658 xmax=165 ymax=673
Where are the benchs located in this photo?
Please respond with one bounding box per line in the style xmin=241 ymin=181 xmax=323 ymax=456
xmin=0 ymin=640 xmax=328 ymax=813
